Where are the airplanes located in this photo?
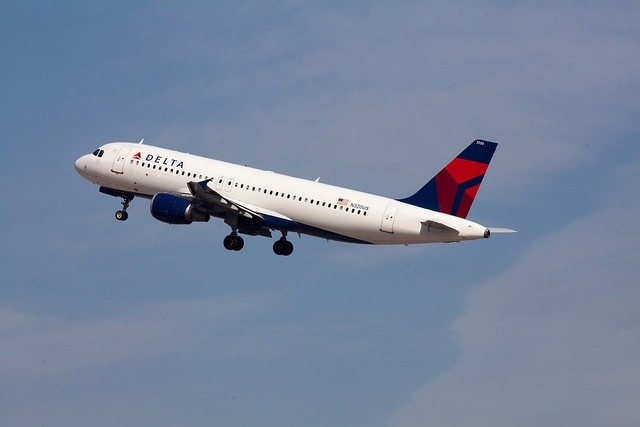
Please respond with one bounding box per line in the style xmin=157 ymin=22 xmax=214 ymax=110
xmin=74 ymin=139 xmax=518 ymax=255
